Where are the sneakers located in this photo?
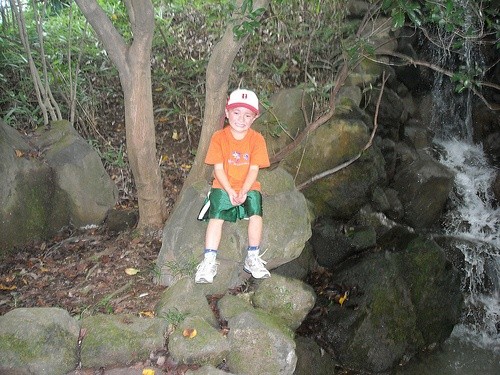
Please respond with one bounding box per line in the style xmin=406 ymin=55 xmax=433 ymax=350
xmin=242 ymin=249 xmax=271 ymax=280
xmin=193 ymin=253 xmax=218 ymax=284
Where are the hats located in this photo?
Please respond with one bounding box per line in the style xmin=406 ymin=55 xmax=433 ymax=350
xmin=226 ymin=89 xmax=259 ymax=115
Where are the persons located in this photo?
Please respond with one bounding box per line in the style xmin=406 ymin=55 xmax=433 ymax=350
xmin=194 ymin=89 xmax=271 ymax=284
